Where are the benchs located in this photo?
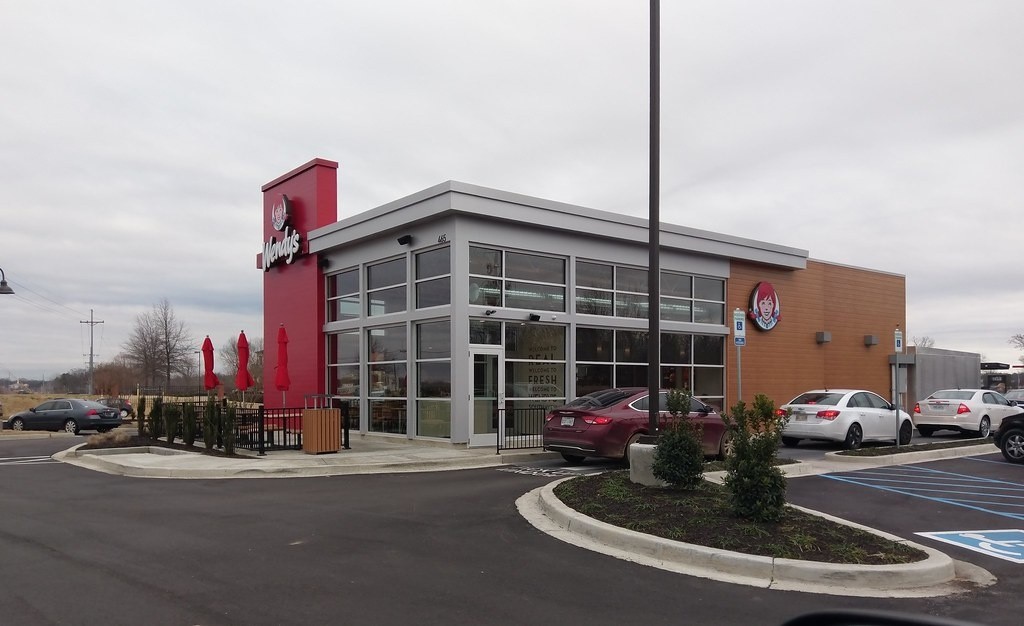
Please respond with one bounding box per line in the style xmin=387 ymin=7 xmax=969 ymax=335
xmin=175 ymin=419 xmax=304 ymax=449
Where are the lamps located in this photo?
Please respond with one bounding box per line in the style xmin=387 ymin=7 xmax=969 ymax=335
xmin=529 ymin=313 xmax=541 ymax=321
xmin=397 ymin=235 xmax=412 ymax=245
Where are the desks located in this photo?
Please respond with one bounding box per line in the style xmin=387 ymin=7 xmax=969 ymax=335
xmin=220 ymin=412 xmax=258 ymax=444
xmin=392 ymin=407 xmax=433 ymax=433
xmin=254 ymin=413 xmax=303 ymax=448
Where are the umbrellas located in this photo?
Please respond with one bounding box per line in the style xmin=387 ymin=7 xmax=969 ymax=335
xmin=274 ymin=322 xmax=291 ymax=408
xmin=201 ymin=335 xmax=220 ymax=401
xmin=234 ymin=330 xmax=255 ymax=408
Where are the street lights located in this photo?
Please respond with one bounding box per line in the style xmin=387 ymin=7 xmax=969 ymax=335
xmin=194 ymin=350 xmax=200 ymax=406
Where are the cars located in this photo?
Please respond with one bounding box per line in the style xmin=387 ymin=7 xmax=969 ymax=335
xmin=6 ymin=399 xmax=121 ymax=436
xmin=913 ymin=389 xmax=1024 ymax=438
xmin=542 ymin=387 xmax=740 ymax=469
xmin=994 ymin=412 xmax=1024 ymax=464
xmin=1003 ymin=389 xmax=1024 ymax=409
xmin=96 ymin=398 xmax=134 ymax=418
xmin=774 ymin=389 xmax=913 ymax=451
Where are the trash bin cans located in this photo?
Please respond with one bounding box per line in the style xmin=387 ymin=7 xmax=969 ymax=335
xmin=302 ymin=393 xmax=341 ymax=455
